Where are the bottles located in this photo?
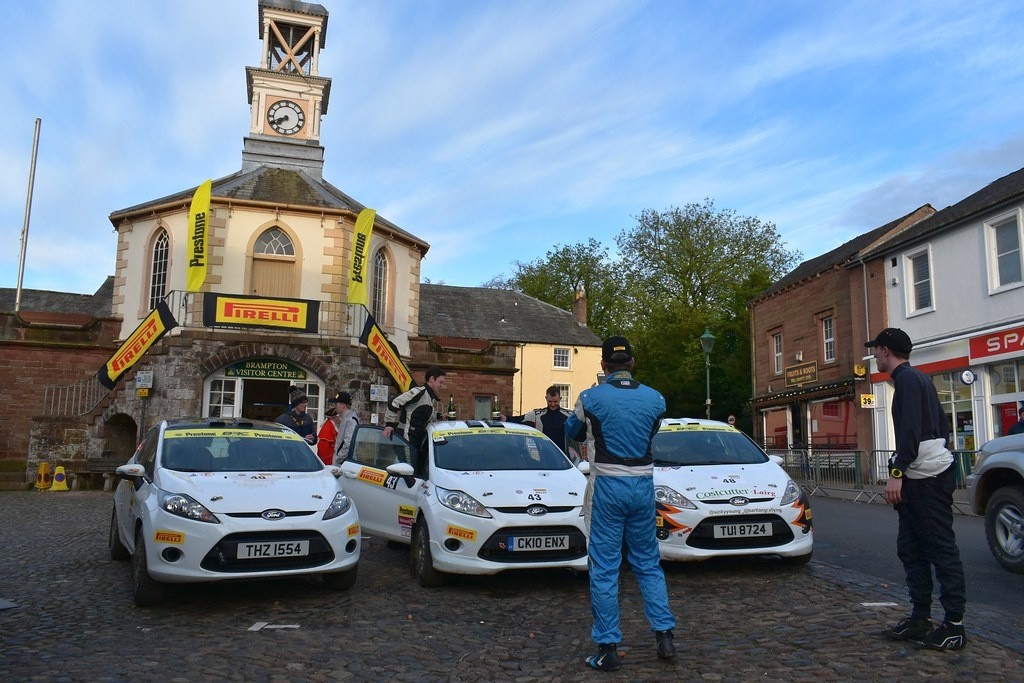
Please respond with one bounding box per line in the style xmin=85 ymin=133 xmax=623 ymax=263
xmin=492 ymin=395 xmax=500 ymax=421
xmin=448 ymin=395 xmax=456 ymax=421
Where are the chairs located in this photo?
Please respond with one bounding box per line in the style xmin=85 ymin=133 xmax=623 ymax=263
xmin=229 ymin=440 xmax=258 ymax=468
xmin=171 ymin=444 xmax=214 ymax=470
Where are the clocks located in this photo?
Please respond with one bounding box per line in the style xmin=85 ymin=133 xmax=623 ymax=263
xmin=267 ymin=99 xmax=305 ymax=135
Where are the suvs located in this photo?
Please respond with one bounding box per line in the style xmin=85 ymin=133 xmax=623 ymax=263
xmin=964 ymin=433 xmax=1023 ymax=572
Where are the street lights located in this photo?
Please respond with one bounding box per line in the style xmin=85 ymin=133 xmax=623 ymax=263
xmin=700 ymin=326 xmax=717 ymax=421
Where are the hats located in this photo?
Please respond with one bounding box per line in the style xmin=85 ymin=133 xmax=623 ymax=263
xmin=865 ymin=328 xmax=912 ymax=353
xmin=328 ymin=392 xmax=353 ymax=404
xmin=288 ymin=385 xmax=308 ymax=408
xmin=602 ymin=336 xmax=632 ymax=363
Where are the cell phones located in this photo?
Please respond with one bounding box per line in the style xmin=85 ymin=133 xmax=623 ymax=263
xmin=597 ymin=373 xmax=605 ymax=386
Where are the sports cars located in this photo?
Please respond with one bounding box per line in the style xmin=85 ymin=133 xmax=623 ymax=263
xmin=107 ymin=416 xmax=360 ymax=603
xmin=651 ymin=418 xmax=812 ymax=571
xmin=337 ymin=418 xmax=591 ymax=588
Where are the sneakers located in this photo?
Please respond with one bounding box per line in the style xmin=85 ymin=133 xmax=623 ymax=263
xmin=887 ymin=616 xmax=934 ymax=640
xmin=585 ymin=643 xmax=620 ymax=671
xmin=655 ymin=630 xmax=676 ymax=658
xmin=914 ymin=621 xmax=967 ymax=650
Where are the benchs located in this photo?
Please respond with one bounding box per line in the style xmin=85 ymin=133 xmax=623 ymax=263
xmin=70 ymin=457 xmax=130 ymax=492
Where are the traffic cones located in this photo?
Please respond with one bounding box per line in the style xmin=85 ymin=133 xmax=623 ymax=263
xmin=34 ymin=462 xmax=51 ymax=488
xmin=49 ymin=466 xmax=69 ymax=491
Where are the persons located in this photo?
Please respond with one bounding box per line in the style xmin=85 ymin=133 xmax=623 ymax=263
xmin=317 ymin=392 xmax=361 ymax=468
xmin=1007 ymin=406 xmax=1024 ymax=435
xmin=382 ymin=365 xmax=449 ymax=475
xmin=275 ymin=385 xmax=318 ymax=446
xmin=864 ymin=328 xmax=967 ymax=650
xmin=565 ymin=336 xmax=679 ymax=670
xmin=500 ymin=385 xmax=573 ymax=459
